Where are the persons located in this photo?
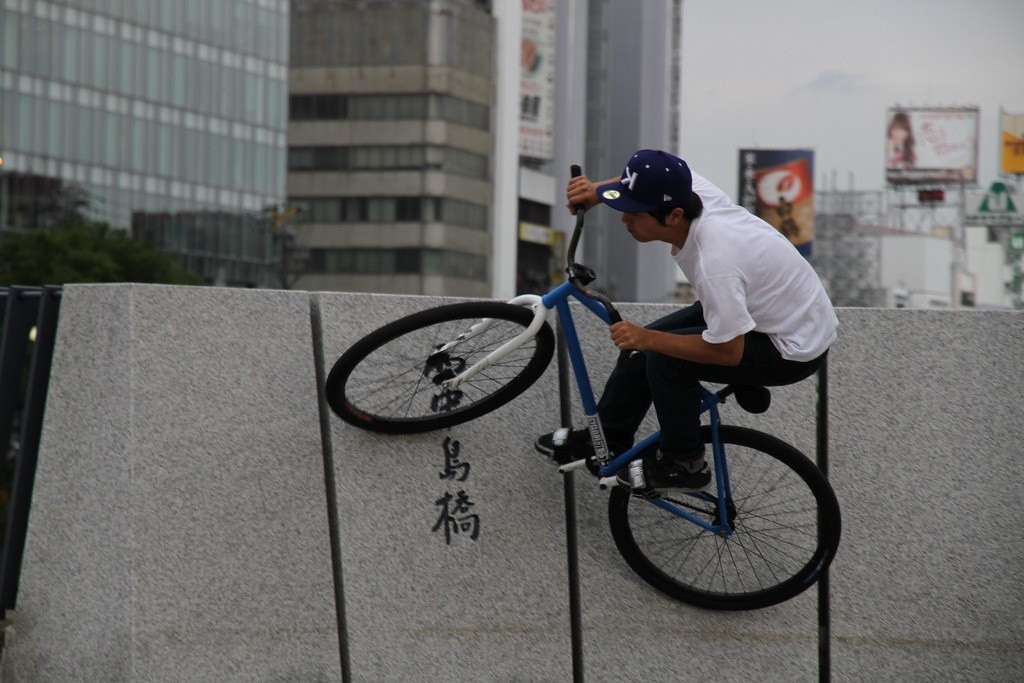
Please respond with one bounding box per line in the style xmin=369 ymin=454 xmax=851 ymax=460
xmin=533 ymin=147 xmax=840 ymax=490
xmin=887 ymin=113 xmax=915 ymax=169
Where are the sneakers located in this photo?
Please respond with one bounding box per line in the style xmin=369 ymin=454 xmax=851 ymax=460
xmin=535 ymin=427 xmax=634 ymax=460
xmin=615 ymin=449 xmax=711 ymax=490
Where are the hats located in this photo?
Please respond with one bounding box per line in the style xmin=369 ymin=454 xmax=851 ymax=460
xmin=596 ymin=149 xmax=692 ymax=212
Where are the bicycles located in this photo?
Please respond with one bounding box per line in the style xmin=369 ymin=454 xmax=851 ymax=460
xmin=325 ymin=160 xmax=844 ymax=613
